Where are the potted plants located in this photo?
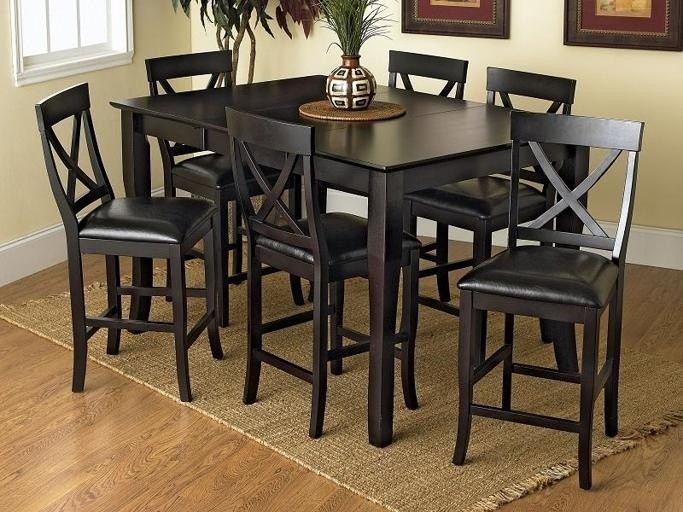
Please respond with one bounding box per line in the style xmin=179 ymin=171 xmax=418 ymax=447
xmin=309 ymin=0 xmax=400 ymax=109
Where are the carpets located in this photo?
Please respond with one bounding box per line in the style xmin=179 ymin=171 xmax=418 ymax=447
xmin=0 ymin=233 xmax=682 ymax=512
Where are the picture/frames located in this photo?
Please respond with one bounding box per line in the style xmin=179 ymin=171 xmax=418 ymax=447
xmin=400 ymin=0 xmax=683 ymax=56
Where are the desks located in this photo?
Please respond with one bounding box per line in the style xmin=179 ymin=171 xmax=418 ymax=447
xmin=106 ymin=73 xmax=597 ymax=447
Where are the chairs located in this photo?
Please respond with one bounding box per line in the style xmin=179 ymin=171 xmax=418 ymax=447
xmin=142 ymin=50 xmax=301 ymax=277
xmin=31 ymin=83 xmax=222 ymax=404
xmin=224 ymin=106 xmax=422 ymax=439
xmin=455 ymin=105 xmax=643 ymax=490
xmin=406 ymin=66 xmax=579 ymax=313
xmin=387 ymin=48 xmax=453 ymax=307
